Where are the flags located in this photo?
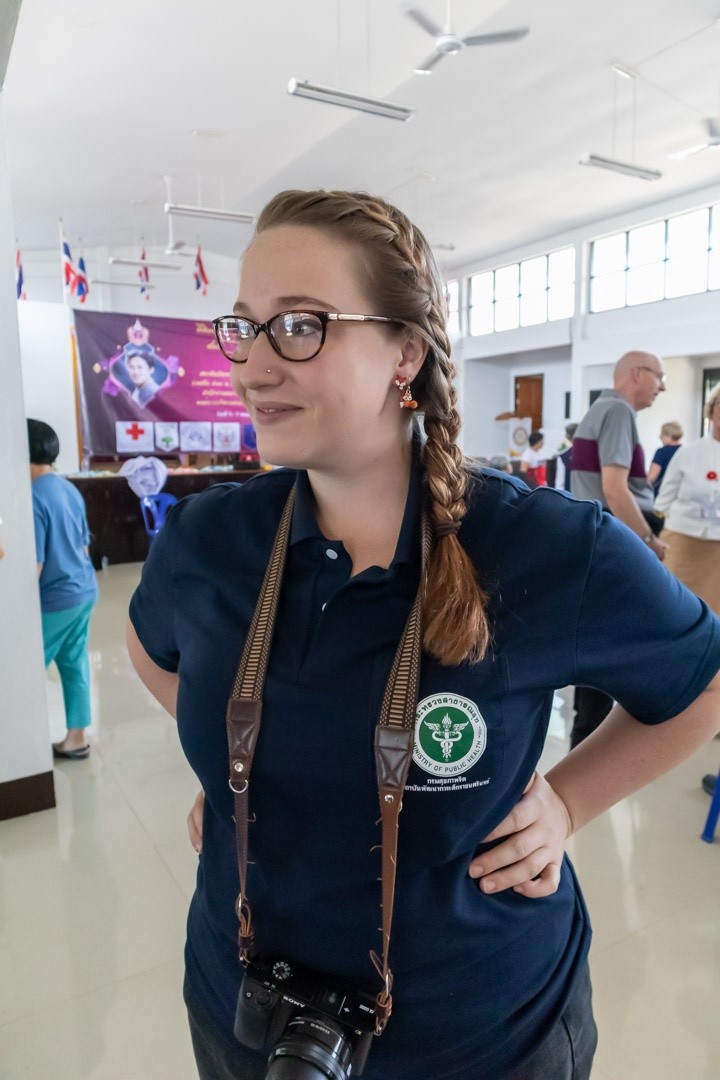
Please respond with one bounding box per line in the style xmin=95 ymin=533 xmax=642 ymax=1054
xmin=61 ymin=237 xmax=90 ymax=303
xmin=139 ymin=246 xmax=150 ymax=299
xmin=193 ymin=245 xmax=209 ymax=296
xmin=15 ymin=250 xmax=26 ymax=301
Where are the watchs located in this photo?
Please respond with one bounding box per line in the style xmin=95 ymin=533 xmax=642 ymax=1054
xmin=643 ymin=530 xmax=654 ymax=543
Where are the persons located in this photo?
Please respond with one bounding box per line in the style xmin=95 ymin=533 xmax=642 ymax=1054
xmin=125 ymin=349 xmax=160 ymax=406
xmin=127 ymin=189 xmax=720 ymax=1080
xmin=569 ymin=350 xmax=668 ymax=752
xmin=487 ymin=383 xmax=720 ymax=621
xmin=27 ymin=418 xmax=97 ymax=759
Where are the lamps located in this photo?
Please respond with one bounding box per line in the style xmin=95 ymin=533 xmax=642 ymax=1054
xmin=109 ymin=226 xmax=183 ymax=270
xmin=90 ymin=241 xmax=155 ymax=288
xmin=287 ymin=0 xmax=415 ymax=122
xmin=578 ymin=63 xmax=660 ymax=180
xmin=163 ymin=171 xmax=256 ymax=225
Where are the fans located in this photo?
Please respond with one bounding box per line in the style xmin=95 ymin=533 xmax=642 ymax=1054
xmin=150 ymin=183 xmax=193 ymax=258
xmin=669 ymin=118 xmax=720 ymax=160
xmin=406 ymin=0 xmax=529 ymax=75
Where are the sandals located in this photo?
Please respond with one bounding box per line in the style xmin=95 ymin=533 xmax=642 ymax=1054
xmin=53 ymin=742 xmax=90 ymax=760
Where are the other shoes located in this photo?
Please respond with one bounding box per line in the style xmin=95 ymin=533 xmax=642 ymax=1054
xmin=702 ymin=773 xmax=718 ymax=796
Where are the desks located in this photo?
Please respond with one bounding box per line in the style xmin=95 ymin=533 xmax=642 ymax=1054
xmin=58 ymin=467 xmax=268 ymax=572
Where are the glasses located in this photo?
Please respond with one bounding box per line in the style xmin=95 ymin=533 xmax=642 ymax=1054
xmin=211 ymin=310 xmax=404 ymax=364
xmin=638 ymin=366 xmax=665 ymax=382
